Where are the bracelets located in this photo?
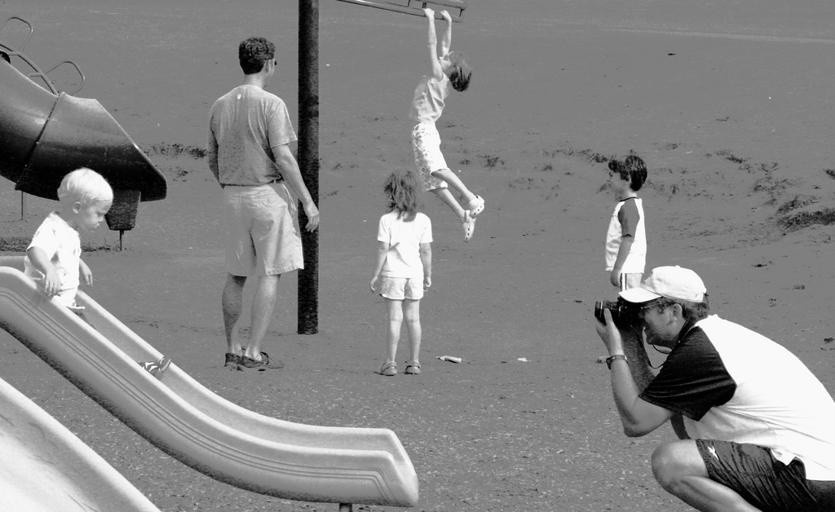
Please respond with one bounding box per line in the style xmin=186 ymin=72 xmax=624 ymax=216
xmin=604 ymin=354 xmax=630 ymax=368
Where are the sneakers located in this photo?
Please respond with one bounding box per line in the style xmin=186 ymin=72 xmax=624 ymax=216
xmin=137 ymin=361 xmax=159 ymax=374
xmin=224 ymin=347 xmax=245 ymax=369
xmin=238 ymin=350 xmax=283 ymax=369
xmin=153 ymin=355 xmax=171 ymax=380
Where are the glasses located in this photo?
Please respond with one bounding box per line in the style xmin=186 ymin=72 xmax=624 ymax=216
xmin=274 ymin=61 xmax=277 ymax=66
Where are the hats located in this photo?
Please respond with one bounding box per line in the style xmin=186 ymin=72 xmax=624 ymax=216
xmin=618 ymin=264 xmax=708 ymax=304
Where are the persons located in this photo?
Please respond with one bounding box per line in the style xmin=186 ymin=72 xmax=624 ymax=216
xmin=605 ymin=152 xmax=648 ymax=297
xmin=371 ymin=168 xmax=435 ymax=375
xmin=411 ymin=9 xmax=485 ymax=241
xmin=23 ymin=168 xmax=171 ymax=377
xmin=593 ymin=264 xmax=834 ymax=510
xmin=207 ymin=37 xmax=319 ymax=372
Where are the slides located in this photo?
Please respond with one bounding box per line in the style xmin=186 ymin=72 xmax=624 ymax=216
xmin=0 ymin=54 xmax=165 ymax=232
xmin=0 ymin=257 xmax=422 ymax=507
xmin=0 ymin=379 xmax=164 ymax=510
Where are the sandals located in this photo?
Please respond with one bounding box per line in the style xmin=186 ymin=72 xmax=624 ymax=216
xmin=462 ymin=210 xmax=477 ymax=242
xmin=404 ymin=359 xmax=422 ymax=374
xmin=469 ymin=194 xmax=486 ymax=217
xmin=380 ymin=361 xmax=399 ymax=375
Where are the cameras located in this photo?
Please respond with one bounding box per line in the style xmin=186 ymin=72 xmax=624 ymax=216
xmin=595 ymin=296 xmax=640 ymax=332
